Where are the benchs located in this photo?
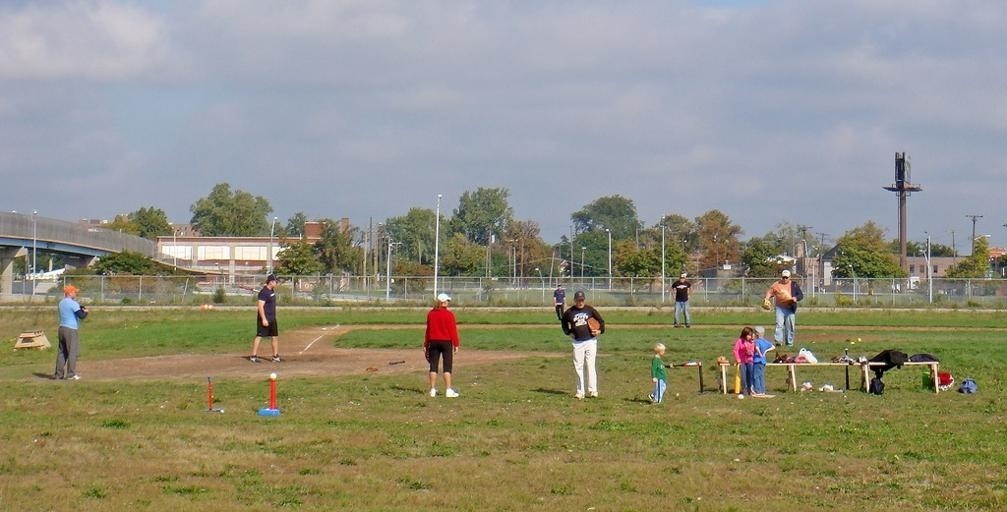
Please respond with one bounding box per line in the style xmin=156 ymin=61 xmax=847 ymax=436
xmin=715 ymin=360 xmax=946 ymax=396
xmin=13 ymin=328 xmax=53 ymax=352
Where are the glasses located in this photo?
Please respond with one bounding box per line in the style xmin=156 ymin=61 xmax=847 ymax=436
xmin=782 ymin=276 xmax=789 ymax=280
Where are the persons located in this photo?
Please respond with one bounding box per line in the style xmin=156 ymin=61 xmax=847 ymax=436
xmin=249 ymin=274 xmax=282 ymax=363
xmin=424 ymin=293 xmax=459 ymax=398
xmin=55 ymin=285 xmax=88 ymax=380
xmin=647 ymin=343 xmax=667 ymax=403
xmin=753 ymin=326 xmax=776 ymax=395
xmin=732 ymin=327 xmax=755 ymax=395
xmin=554 ymin=284 xmax=564 ymax=320
xmin=672 ymin=272 xmax=690 ymax=328
xmin=763 ymin=270 xmax=803 ymax=347
xmin=562 ymin=291 xmax=605 ymax=400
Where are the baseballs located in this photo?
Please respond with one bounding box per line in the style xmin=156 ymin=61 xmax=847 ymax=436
xmin=270 ymin=373 xmax=277 ymax=380
xmin=738 ymin=394 xmax=744 ymax=399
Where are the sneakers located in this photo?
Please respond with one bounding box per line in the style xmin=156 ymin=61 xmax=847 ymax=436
xmin=446 ymin=388 xmax=460 ymax=397
xmin=646 ymin=393 xmax=655 ymax=402
xmin=586 ymin=391 xmax=598 ymax=398
xmin=430 ymin=389 xmax=438 ymax=398
xmin=574 ymin=393 xmax=585 ymax=399
xmin=272 ymin=354 xmax=285 ymax=363
xmin=67 ymin=374 xmax=81 ymax=381
xmin=249 ymin=355 xmax=262 ymax=363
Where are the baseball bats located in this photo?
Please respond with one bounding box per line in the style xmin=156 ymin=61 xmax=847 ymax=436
xmin=845 ymin=349 xmax=849 ymax=390
xmin=663 ymin=362 xmax=701 ymax=368
xmin=735 ymin=363 xmax=740 ymax=393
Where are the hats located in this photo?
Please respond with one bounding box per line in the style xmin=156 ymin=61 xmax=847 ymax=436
xmin=782 ymin=270 xmax=792 ymax=277
xmin=575 ymin=291 xmax=586 ymax=301
xmin=267 ymin=274 xmax=280 ymax=281
xmin=64 ymin=285 xmax=80 ymax=295
xmin=438 ymin=293 xmax=452 ymax=303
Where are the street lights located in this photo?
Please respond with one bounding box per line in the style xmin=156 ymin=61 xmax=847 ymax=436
xmin=31 ymin=209 xmax=38 ymax=296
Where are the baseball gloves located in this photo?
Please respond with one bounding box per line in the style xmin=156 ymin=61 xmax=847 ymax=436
xmin=588 ymin=317 xmax=600 ymax=330
xmin=762 ymin=299 xmax=771 ymax=310
